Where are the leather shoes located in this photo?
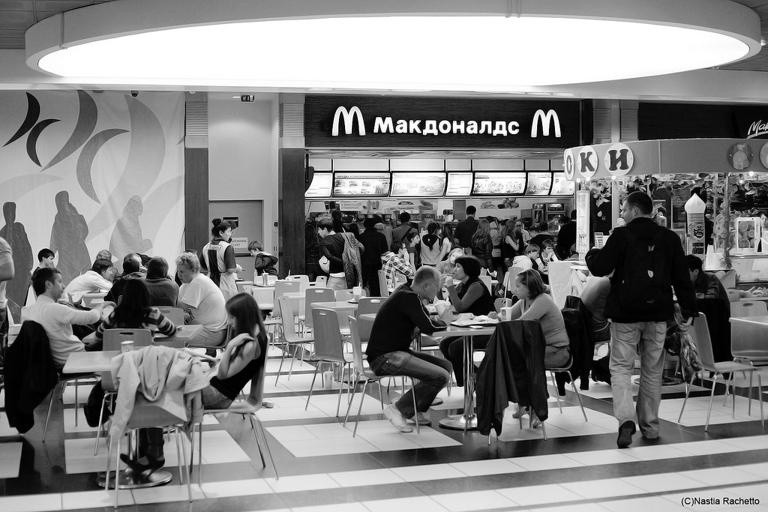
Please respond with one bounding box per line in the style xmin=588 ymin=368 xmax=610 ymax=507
xmin=618 ymin=420 xmax=636 ymax=448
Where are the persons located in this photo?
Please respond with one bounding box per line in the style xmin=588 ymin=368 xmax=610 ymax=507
xmin=200 ymin=218 xmax=242 ymax=302
xmin=121 ymin=292 xmax=267 ymax=481
xmin=306 ymin=202 xmax=768 ymax=449
xmin=1 ymin=238 xmax=15 ymax=331
xmin=248 ymin=240 xmax=278 ymax=320
xmin=21 ymin=248 xmax=229 ymax=377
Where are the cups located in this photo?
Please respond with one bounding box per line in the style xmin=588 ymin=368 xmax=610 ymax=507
xmin=122 ymin=341 xmax=134 ymax=353
xmin=500 ymin=308 xmax=512 ymax=322
xmin=352 ymin=287 xmax=362 ymax=303
xmin=262 ymin=273 xmax=269 ymax=285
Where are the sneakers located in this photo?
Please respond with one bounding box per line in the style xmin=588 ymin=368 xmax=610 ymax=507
xmin=513 ymin=407 xmax=541 ymax=428
xmin=383 ymin=400 xmax=431 ymax=432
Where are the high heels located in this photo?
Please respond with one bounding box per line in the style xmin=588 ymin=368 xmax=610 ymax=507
xmin=120 ymin=454 xmax=164 ymax=478
